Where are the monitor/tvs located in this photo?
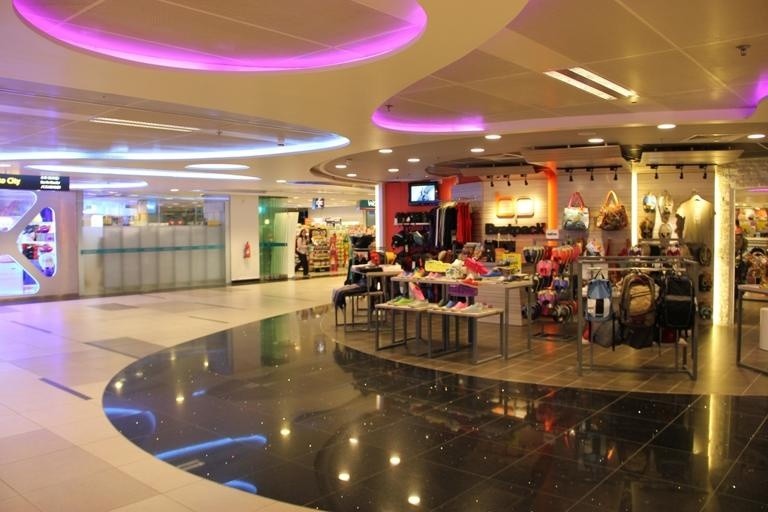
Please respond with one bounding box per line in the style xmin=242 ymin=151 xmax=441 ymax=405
xmin=408 ymin=181 xmax=440 ymax=206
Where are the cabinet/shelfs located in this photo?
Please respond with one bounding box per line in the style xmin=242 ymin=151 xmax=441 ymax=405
xmin=332 ymin=262 xmax=534 ymax=365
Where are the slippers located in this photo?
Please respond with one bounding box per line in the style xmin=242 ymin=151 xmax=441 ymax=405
xmin=521 ymin=245 xmax=575 ymax=324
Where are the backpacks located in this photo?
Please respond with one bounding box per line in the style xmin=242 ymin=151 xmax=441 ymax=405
xmin=584 ymin=270 xmax=615 ymax=322
xmin=659 ymin=275 xmax=695 ymax=329
xmin=595 ymin=319 xmax=680 ymax=348
xmin=618 ymin=271 xmax=660 ymax=328
xmin=294 ymin=237 xmax=304 ymax=255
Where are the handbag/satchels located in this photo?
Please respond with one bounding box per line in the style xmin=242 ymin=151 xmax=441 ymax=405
xmin=562 ymin=191 xmax=591 ymax=231
xmin=596 ymin=191 xmax=629 ymax=232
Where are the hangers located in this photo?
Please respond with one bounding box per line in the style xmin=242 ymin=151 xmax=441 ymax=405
xmin=678 ymin=193 xmax=715 ymax=215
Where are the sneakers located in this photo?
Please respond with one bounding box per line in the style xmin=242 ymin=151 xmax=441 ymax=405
xmin=303 ymin=273 xmax=311 ymax=278
xmin=387 ymin=300 xmax=483 ymax=313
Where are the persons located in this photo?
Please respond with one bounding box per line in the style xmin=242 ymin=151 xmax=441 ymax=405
xmin=420 ymin=186 xmax=428 ymax=201
xmin=380 ymin=238 xmax=413 ymax=312
xmin=295 ymin=228 xmax=310 ymax=277
xmin=732 ymin=216 xmax=748 ymax=301
